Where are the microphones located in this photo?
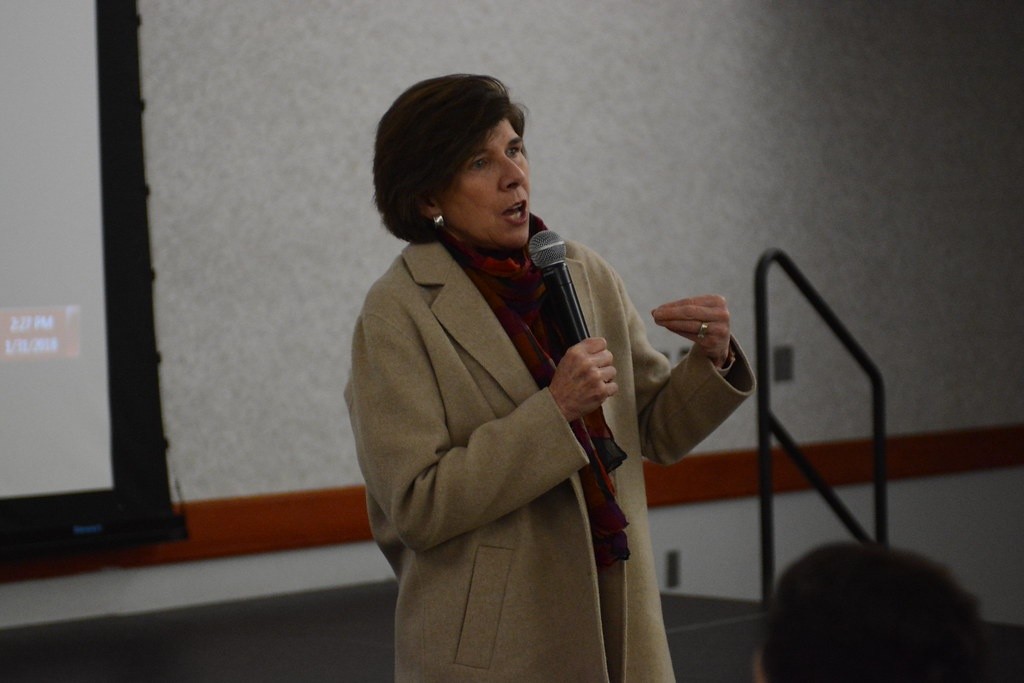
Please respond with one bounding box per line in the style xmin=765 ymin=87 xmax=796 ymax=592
xmin=529 ymin=229 xmax=592 ymax=344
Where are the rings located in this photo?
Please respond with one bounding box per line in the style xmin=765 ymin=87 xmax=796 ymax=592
xmin=696 ymin=323 xmax=710 ymax=340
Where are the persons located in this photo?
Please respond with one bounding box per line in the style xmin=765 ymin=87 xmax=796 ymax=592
xmin=750 ymin=541 xmax=989 ymax=683
xmin=344 ymin=70 xmax=755 ymax=683
